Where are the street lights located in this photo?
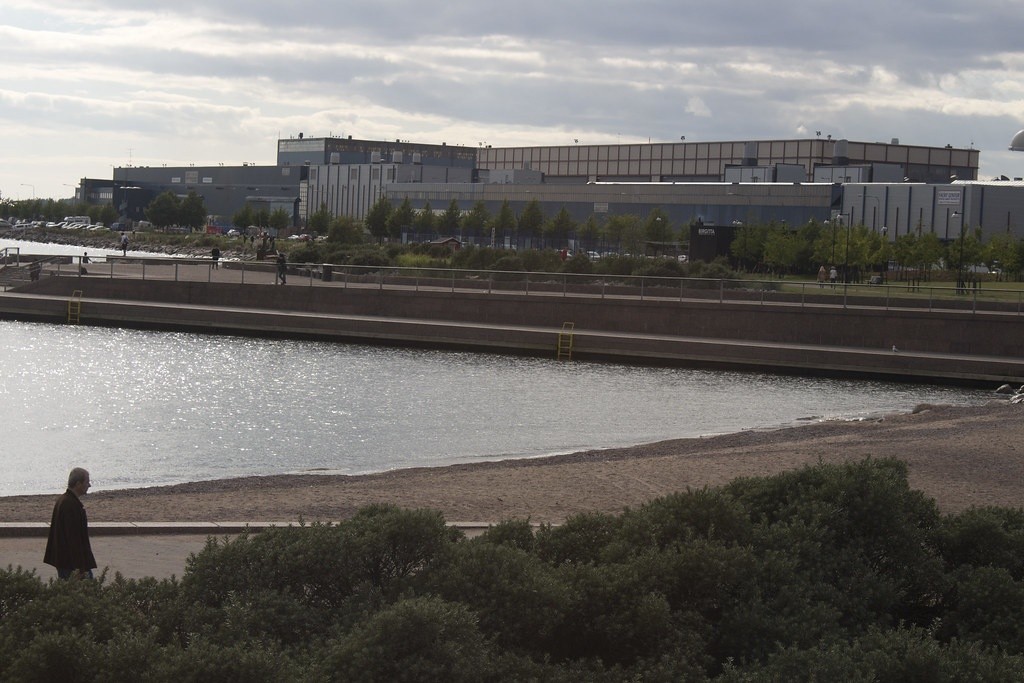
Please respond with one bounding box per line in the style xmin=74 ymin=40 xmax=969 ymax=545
xmin=730 ymin=218 xmax=747 ymax=269
xmin=655 ymin=216 xmax=665 ymax=258
xmin=836 ymin=212 xmax=851 ymax=309
xmin=62 ymin=183 xmax=77 ymax=216
xmin=856 ymin=193 xmax=880 ymax=235
xmin=950 ymin=185 xmax=967 ymax=293
xmin=19 ymin=183 xmax=36 ymax=199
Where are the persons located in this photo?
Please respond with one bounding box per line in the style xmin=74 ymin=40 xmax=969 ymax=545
xmin=276 ymin=250 xmax=287 ymax=285
xmin=82 ymin=252 xmax=92 ymax=264
xmin=830 ymin=266 xmax=838 ymax=288
xmin=43 ymin=467 xmax=97 ymax=579
xmin=817 ymin=266 xmax=826 ymax=288
xmin=211 ymin=246 xmax=220 ymax=269
xmin=29 ymin=259 xmax=41 ymax=282
xmin=121 ymin=233 xmax=129 ymax=256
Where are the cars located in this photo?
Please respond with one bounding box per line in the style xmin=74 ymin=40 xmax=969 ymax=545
xmin=289 ymin=233 xmax=313 ymax=241
xmin=556 ymin=249 xmax=620 ymax=264
xmin=227 ymin=228 xmax=241 ymax=236
xmin=0 ymin=217 xmax=112 ymax=231
